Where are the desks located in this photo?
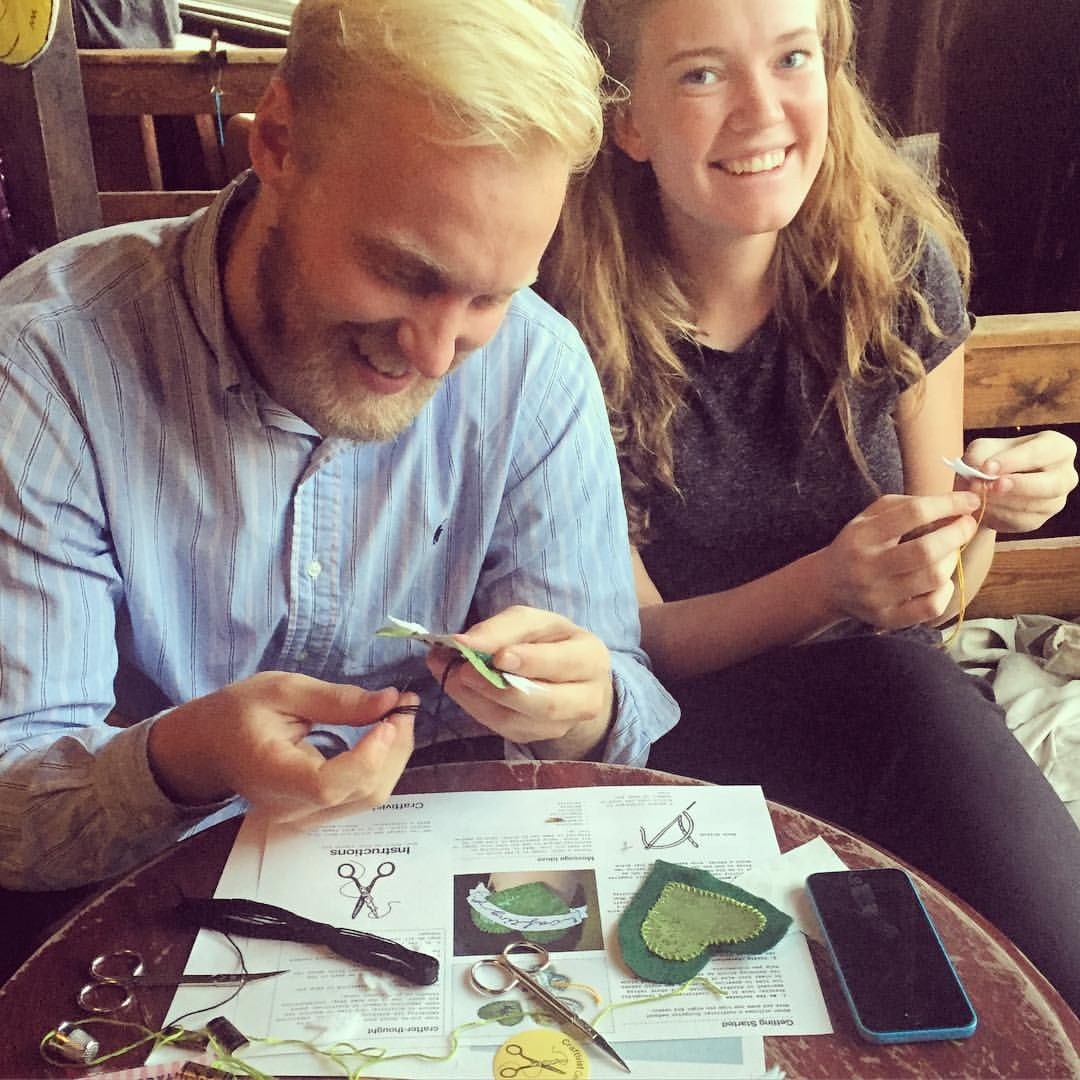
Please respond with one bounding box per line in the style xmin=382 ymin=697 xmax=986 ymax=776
xmin=0 ymin=761 xmax=1080 ymax=1079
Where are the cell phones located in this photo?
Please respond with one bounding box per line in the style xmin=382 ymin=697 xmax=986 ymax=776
xmin=805 ymin=867 xmax=979 ymax=1044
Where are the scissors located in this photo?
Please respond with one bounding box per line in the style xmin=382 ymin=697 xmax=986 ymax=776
xmin=498 ymin=1043 xmax=568 ymax=1079
xmin=336 ymin=862 xmax=395 ymax=919
xmin=77 ymin=949 xmax=291 ymax=1014
xmin=469 ymin=941 xmax=630 ymax=1072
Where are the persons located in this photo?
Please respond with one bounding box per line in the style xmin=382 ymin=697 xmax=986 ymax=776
xmin=532 ymin=0 xmax=1079 ymax=1018
xmin=0 ymin=0 xmax=682 ymax=994
